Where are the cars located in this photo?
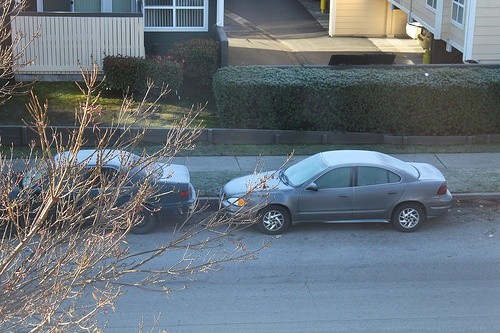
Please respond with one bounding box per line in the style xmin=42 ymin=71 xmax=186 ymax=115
xmin=1 ymin=149 xmax=196 ymax=234
xmin=218 ymin=150 xmax=453 ymax=235
